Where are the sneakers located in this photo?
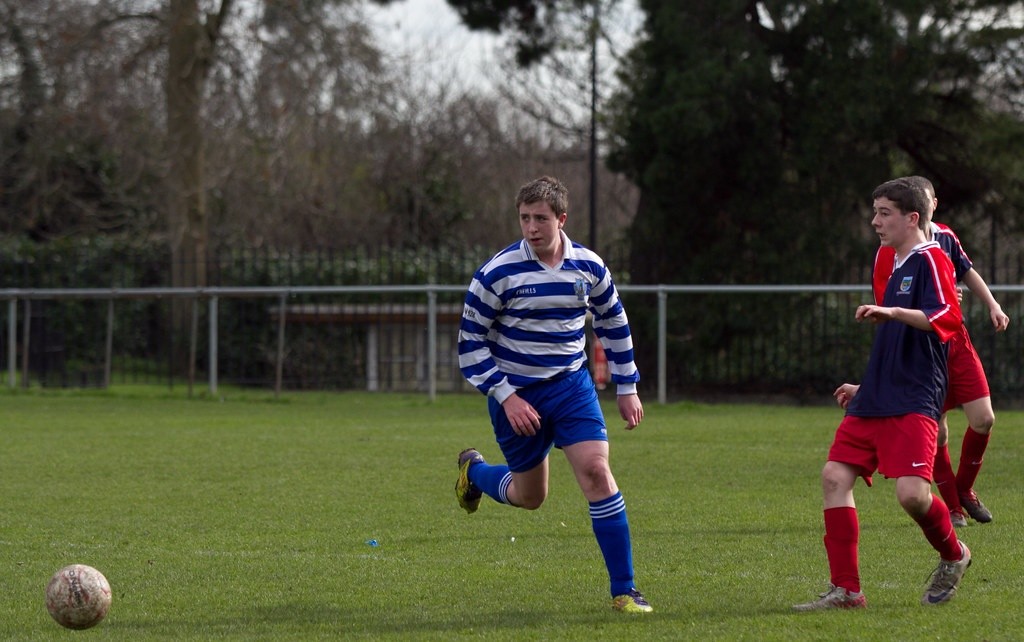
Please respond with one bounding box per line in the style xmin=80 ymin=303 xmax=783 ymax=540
xmin=948 ymin=509 xmax=968 ymax=527
xmin=455 ymin=448 xmax=483 ymax=514
xmin=920 ymin=541 xmax=971 ymax=606
xmin=959 ymin=490 xmax=992 ymax=523
xmin=612 ymin=589 xmax=651 ymax=614
xmin=793 ymin=584 xmax=866 ymax=612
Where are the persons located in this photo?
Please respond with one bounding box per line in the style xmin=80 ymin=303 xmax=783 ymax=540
xmin=788 ymin=175 xmax=973 ymax=614
xmin=873 ymin=176 xmax=1012 ymax=527
xmin=455 ymin=175 xmax=655 ymax=614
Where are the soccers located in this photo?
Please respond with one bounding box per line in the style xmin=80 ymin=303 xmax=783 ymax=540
xmin=46 ymin=563 xmax=113 ymax=631
